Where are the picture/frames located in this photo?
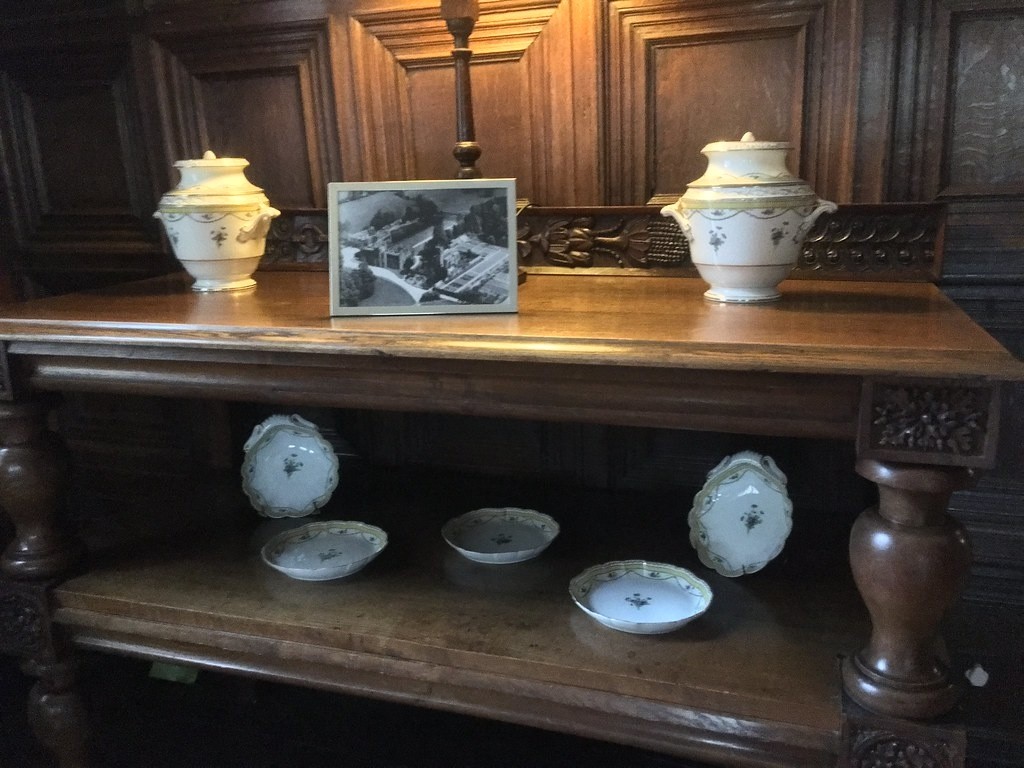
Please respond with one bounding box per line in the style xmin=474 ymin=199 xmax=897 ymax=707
xmin=327 ymin=178 xmax=519 ymax=317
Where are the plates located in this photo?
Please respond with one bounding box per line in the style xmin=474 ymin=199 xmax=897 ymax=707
xmin=688 ymin=450 xmax=796 ymax=578
xmin=568 ymin=559 xmax=712 ymax=635
xmin=239 ymin=412 xmax=338 ymax=517
xmin=441 ymin=507 xmax=559 ymax=563
xmin=260 ymin=520 xmax=388 ymax=581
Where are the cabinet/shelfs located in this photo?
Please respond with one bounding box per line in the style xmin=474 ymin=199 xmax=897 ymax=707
xmin=0 ymin=270 xmax=1024 ymax=768
